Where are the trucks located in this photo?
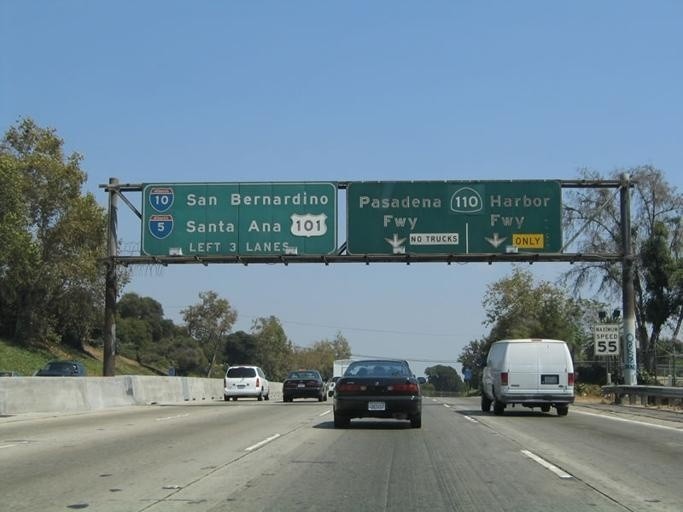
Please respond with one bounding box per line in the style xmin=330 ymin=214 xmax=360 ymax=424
xmin=479 ymin=337 xmax=577 ymax=417
xmin=331 ymin=359 xmax=353 ymax=377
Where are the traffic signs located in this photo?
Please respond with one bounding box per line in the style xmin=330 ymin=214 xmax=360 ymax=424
xmin=344 ymin=179 xmax=563 ymax=259
xmin=593 ymin=323 xmax=620 ymax=356
xmin=137 ymin=179 xmax=340 ymax=261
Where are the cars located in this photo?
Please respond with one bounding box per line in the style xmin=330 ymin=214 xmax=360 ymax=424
xmin=34 ymin=358 xmax=85 ymax=377
xmin=332 ymin=357 xmax=428 ymax=430
xmin=222 ymin=364 xmax=343 ymax=402
